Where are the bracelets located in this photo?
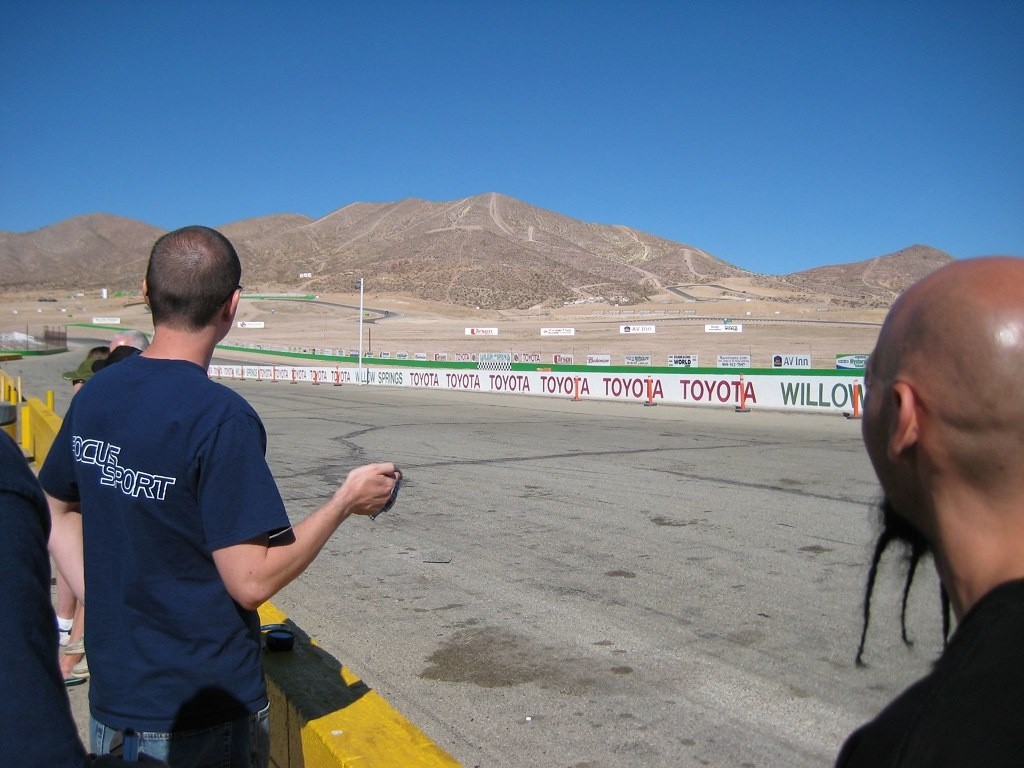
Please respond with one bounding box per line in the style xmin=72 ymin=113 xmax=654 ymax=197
xmin=369 ymin=467 xmax=403 ymax=520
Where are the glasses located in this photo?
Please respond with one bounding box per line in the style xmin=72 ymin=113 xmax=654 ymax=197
xmin=73 ymin=379 xmax=85 ymax=385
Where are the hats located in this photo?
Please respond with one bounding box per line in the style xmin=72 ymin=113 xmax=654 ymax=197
xmin=92 ymin=346 xmax=144 ymax=373
xmin=63 ymin=356 xmax=104 ymax=382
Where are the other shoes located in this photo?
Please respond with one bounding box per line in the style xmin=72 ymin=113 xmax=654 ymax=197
xmin=70 ymin=654 xmax=90 ymax=678
xmin=59 ymin=632 xmax=70 ymax=645
xmin=64 ymin=634 xmax=86 ymax=654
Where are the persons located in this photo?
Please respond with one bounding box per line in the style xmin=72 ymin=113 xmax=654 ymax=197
xmin=1 ymin=224 xmax=404 ymax=768
xmin=834 ymin=252 xmax=1024 ymax=768
